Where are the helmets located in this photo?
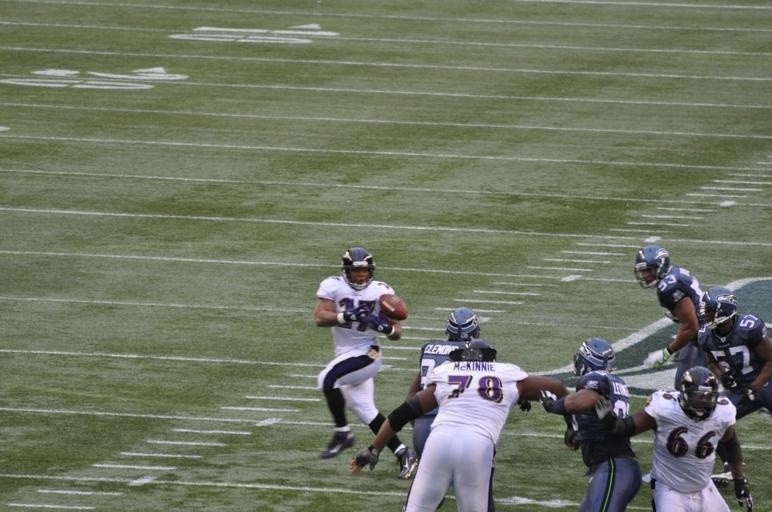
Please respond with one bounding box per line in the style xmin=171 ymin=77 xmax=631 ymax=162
xmin=680 ymin=366 xmax=719 ymax=418
xmin=448 ymin=307 xmax=479 ymax=342
xmin=694 ymin=286 xmax=738 ymax=332
xmin=634 ymin=246 xmax=670 ymax=289
xmin=341 ymin=248 xmax=376 ymax=291
xmin=460 ymin=338 xmax=493 ymax=362
xmin=573 ymin=337 xmax=616 ymax=376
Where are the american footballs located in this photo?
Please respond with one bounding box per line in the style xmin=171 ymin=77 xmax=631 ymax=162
xmin=379 ymin=294 xmax=408 ymax=320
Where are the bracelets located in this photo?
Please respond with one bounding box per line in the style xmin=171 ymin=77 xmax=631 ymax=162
xmin=387 ymin=325 xmax=395 ymax=336
xmin=337 ymin=312 xmax=346 ymax=324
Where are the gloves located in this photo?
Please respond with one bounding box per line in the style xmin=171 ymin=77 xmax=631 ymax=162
xmin=539 ymin=390 xmax=557 ymax=412
xmin=643 ymin=346 xmax=672 ymax=370
xmin=731 ymin=477 xmax=753 ymax=512
xmin=734 ymin=385 xmax=765 ymax=409
xmin=336 ymin=304 xmax=395 ymax=337
xmin=720 ymin=370 xmax=742 ymax=391
xmin=517 ymin=399 xmax=531 ymax=412
xmin=593 ymin=395 xmax=617 ymax=430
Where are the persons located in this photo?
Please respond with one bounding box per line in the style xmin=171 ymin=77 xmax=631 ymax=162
xmin=314 ymin=247 xmax=419 ymax=480
xmin=537 ymin=337 xmax=642 ymax=512
xmin=406 ymin=306 xmax=532 ymax=512
xmin=696 ymin=287 xmax=772 ymax=486
xmin=594 ymin=366 xmax=753 ymax=512
xmin=349 ymin=340 xmax=579 ymax=512
xmin=633 ymin=246 xmax=709 ymax=390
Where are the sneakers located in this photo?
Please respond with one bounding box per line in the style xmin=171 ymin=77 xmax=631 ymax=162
xmin=321 ymin=431 xmax=355 ymax=458
xmin=710 ymin=471 xmax=747 ymax=488
xmin=398 ymin=449 xmax=418 ymax=479
